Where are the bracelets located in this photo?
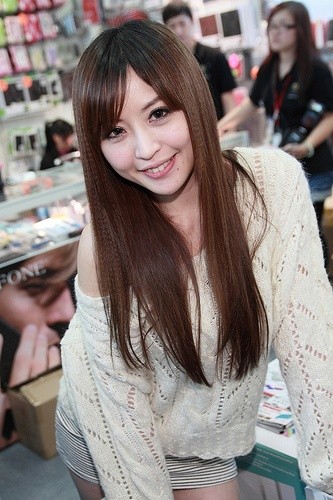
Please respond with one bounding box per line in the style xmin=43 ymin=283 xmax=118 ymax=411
xmin=302 ymin=141 xmax=314 ymax=158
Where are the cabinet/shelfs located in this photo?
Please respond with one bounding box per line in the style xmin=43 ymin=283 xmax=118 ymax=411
xmin=0 ymin=132 xmax=249 ymax=268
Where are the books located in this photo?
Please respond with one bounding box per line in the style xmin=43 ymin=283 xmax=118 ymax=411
xmin=256 ymin=358 xmax=296 ymax=437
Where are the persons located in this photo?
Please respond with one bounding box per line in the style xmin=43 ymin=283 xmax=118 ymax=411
xmin=36 ymin=119 xmax=79 ymax=220
xmin=215 ymin=1 xmax=333 ymax=282
xmin=163 ymin=0 xmax=237 ymax=132
xmin=55 ymin=19 xmax=333 ymax=500
xmin=0 ymin=240 xmax=79 ymax=389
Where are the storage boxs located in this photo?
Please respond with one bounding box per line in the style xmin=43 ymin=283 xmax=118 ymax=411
xmin=8 ymin=365 xmax=67 ymax=459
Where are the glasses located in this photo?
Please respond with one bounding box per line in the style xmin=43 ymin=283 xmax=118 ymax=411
xmin=268 ymin=22 xmax=296 ymax=32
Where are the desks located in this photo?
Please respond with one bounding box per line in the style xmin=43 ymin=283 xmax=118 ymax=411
xmin=235 ymin=358 xmax=309 ymax=500
